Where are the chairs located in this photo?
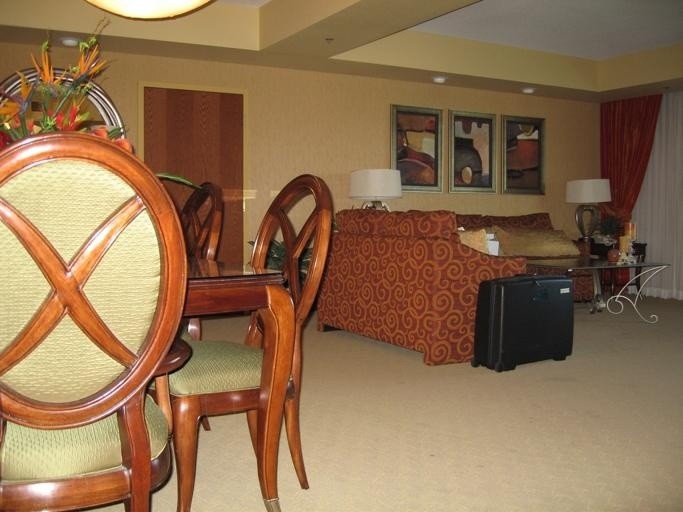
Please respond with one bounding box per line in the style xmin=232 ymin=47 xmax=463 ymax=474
xmin=146 ymin=175 xmax=333 ymax=511
xmin=178 ymin=183 xmax=225 ymax=433
xmin=0 ymin=135 xmax=193 ymax=512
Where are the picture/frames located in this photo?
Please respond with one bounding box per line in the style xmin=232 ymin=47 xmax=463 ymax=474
xmin=390 ymin=102 xmax=546 ymax=195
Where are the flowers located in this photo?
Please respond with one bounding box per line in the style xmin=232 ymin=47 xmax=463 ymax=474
xmin=0 ymin=16 xmax=207 ymax=190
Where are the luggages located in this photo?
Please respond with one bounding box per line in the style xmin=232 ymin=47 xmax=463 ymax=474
xmin=471 ymin=272 xmax=575 ymax=373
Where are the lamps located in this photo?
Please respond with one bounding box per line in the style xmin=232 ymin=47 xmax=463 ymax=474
xmin=350 ymin=168 xmax=402 ymax=210
xmin=564 ymin=179 xmax=612 ymax=243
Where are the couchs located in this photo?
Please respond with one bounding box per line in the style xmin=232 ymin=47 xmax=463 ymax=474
xmin=316 ymin=210 xmax=596 ymax=368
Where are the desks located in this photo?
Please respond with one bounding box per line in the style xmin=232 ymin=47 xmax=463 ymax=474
xmin=181 ymin=259 xmax=297 ymax=511
xmin=590 ymin=241 xmax=647 ymax=303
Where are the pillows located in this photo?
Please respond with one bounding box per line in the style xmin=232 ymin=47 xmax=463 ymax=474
xmin=459 ymin=224 xmax=581 ymax=259
